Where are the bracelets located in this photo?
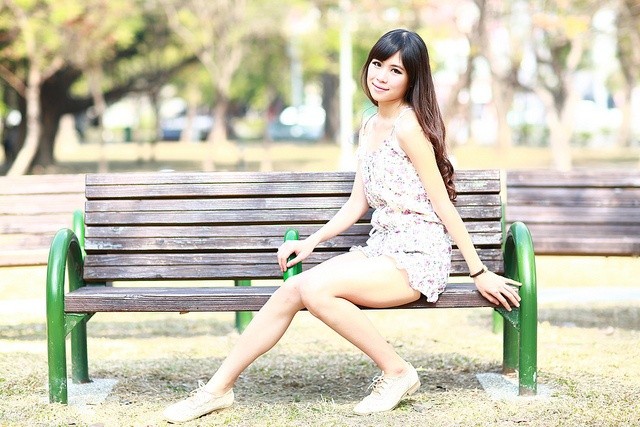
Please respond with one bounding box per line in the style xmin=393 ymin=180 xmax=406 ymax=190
xmin=469 ymin=265 xmax=488 ymax=278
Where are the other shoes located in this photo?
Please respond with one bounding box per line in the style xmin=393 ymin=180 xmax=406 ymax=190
xmin=162 ymin=379 xmax=236 ymax=422
xmin=353 ymin=361 xmax=421 ymax=417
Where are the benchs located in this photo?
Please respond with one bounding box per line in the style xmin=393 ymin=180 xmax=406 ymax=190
xmin=0 ymin=175 xmax=253 ymax=333
xmin=493 ymin=170 xmax=640 ymax=337
xmin=46 ymin=169 xmax=538 ymax=404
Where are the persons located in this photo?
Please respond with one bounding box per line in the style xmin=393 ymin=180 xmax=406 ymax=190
xmin=162 ymin=28 xmax=522 ymax=425
